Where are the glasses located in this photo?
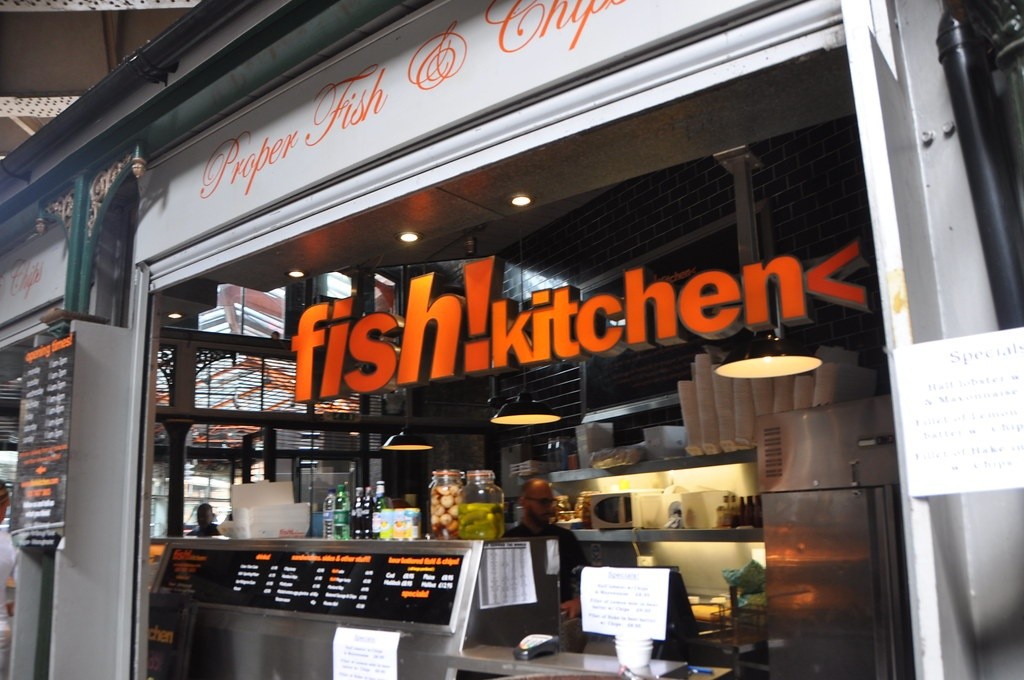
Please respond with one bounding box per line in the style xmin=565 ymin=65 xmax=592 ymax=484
xmin=525 ymin=496 xmax=554 ymax=506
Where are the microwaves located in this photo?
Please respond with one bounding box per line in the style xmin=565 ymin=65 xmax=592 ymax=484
xmin=591 ymin=488 xmax=666 ymax=528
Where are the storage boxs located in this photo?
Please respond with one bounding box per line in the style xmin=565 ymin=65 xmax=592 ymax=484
xmin=643 ymin=425 xmax=686 ymax=459
xmin=575 ymin=423 xmax=613 ymax=468
xmin=231 ymin=480 xmax=311 ymax=540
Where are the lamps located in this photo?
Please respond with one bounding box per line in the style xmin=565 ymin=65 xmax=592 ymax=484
xmin=490 ymin=228 xmax=563 ymax=426
xmin=712 ymin=330 xmax=823 ymax=379
xmin=380 ymin=415 xmax=434 ymax=451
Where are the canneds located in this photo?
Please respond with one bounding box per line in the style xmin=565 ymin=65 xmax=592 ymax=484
xmin=379 ymin=508 xmax=422 ymax=539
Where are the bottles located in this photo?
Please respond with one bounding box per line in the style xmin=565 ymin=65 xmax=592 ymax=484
xmin=717 ymin=496 xmax=762 ymax=528
xmin=431 ymin=470 xmax=464 ymax=539
xmin=323 ymin=489 xmax=335 ymax=539
xmin=546 ymin=435 xmax=577 ymax=472
xmin=333 ymin=485 xmax=351 ymax=540
xmin=362 ymin=487 xmax=373 ymax=539
xmin=344 ymin=481 xmax=351 ymax=511
xmin=459 ymin=470 xmax=505 ymax=540
xmin=352 ymin=487 xmax=365 ymax=539
xmin=371 ymin=481 xmax=387 ymax=539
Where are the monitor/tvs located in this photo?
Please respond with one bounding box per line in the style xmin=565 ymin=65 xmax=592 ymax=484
xmin=571 ymin=565 xmax=699 ymax=659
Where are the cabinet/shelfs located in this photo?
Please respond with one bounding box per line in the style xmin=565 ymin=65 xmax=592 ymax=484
xmin=518 ymin=448 xmax=764 ymax=542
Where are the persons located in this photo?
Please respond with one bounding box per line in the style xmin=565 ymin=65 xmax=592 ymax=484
xmin=501 ymin=477 xmax=590 ymax=618
xmin=0 ymin=479 xmax=21 ymax=680
xmin=187 ymin=503 xmax=226 ymax=537
